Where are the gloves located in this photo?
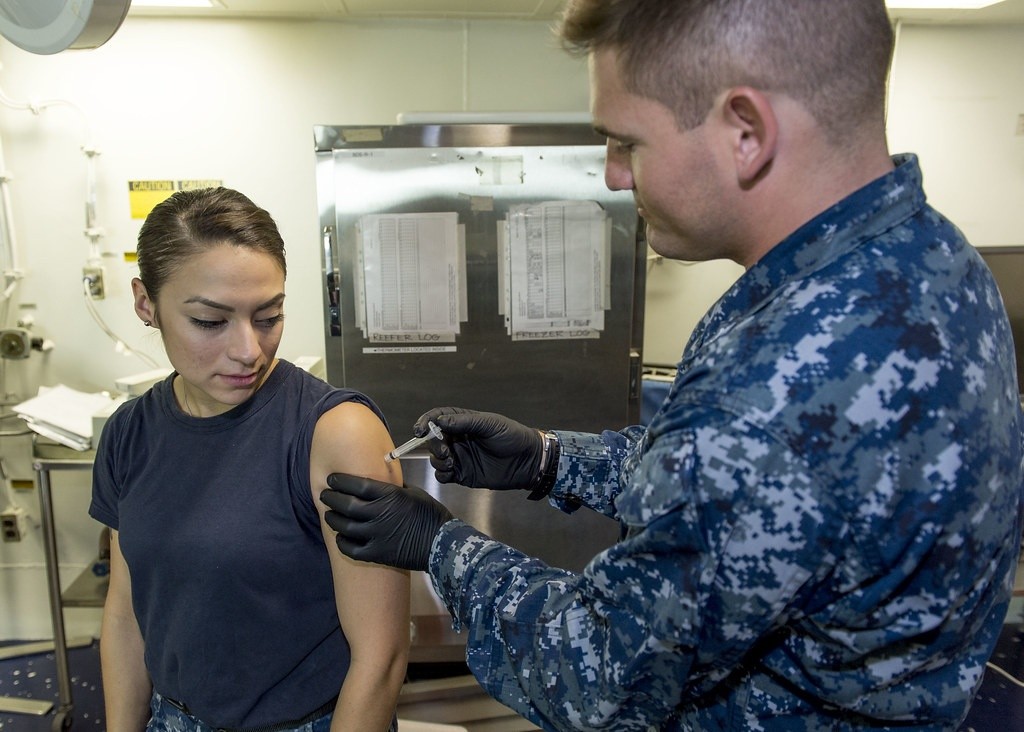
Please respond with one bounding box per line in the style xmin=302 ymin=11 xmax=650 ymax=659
xmin=320 ymin=472 xmax=455 ymax=574
xmin=412 ymin=404 xmax=542 ymax=492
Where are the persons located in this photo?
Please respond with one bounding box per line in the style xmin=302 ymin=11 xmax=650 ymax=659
xmin=318 ymin=1 xmax=1023 ymax=732
xmin=86 ymin=185 xmax=408 ymax=732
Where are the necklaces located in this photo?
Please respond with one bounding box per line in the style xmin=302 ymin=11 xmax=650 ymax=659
xmin=182 ymin=377 xmax=195 ymax=419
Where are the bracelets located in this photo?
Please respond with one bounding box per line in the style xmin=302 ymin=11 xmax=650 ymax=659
xmin=526 ymin=428 xmax=561 ymax=502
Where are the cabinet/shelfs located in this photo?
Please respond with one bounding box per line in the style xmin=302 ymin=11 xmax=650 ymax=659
xmin=30 ymin=432 xmax=109 ymax=705
